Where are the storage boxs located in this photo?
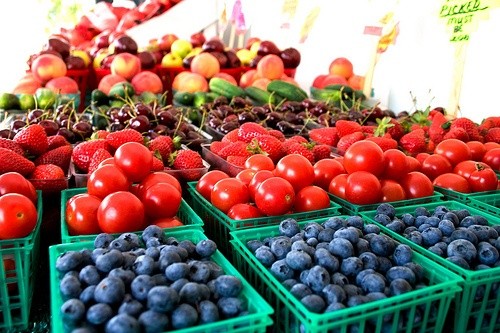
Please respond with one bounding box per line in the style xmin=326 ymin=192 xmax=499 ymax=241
xmin=0 ymin=109 xmax=500 ymax=333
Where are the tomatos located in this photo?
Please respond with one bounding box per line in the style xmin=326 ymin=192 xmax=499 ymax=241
xmin=0 ymin=138 xmax=500 ymax=241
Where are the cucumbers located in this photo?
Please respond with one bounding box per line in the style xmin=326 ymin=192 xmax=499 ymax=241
xmin=0 ymin=77 xmax=367 ymax=109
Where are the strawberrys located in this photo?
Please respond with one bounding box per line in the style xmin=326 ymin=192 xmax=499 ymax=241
xmin=0 ymin=118 xmax=500 ymax=190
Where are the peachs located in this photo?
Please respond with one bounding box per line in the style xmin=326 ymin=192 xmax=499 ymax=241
xmin=12 ymin=52 xmax=365 ymax=95
